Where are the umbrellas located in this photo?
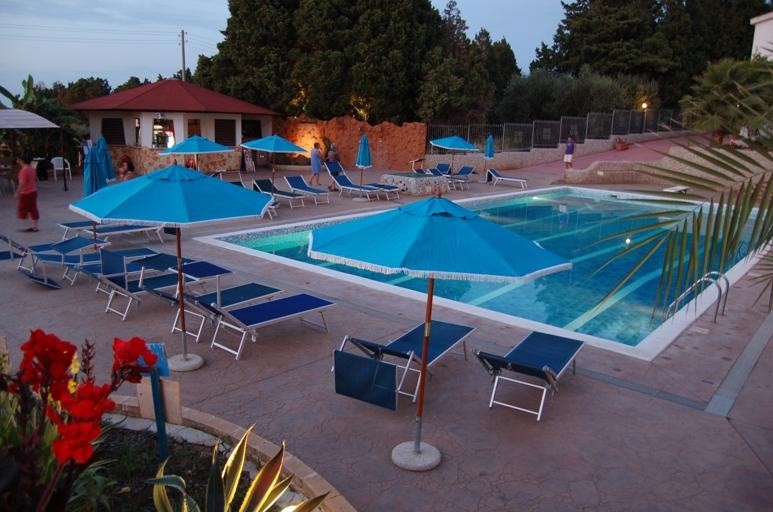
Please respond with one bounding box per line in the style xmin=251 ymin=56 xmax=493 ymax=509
xmin=95 ymin=131 xmax=118 ymax=185
xmin=83 ymin=143 xmax=108 ymax=251
xmin=239 ymin=133 xmax=309 ymax=196
xmin=429 ymin=135 xmax=480 ymax=176
xmin=305 ymin=188 xmax=573 ymax=454
xmin=483 ymin=134 xmax=496 ymax=177
xmin=67 ymin=158 xmax=275 ymax=362
xmin=355 ymin=134 xmax=373 ymax=188
xmin=157 ymin=133 xmax=236 ymax=173
xmin=0 ymin=108 xmax=61 ymax=129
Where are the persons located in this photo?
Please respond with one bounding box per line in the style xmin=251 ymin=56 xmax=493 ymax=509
xmin=14 ymin=148 xmax=40 ymax=233
xmin=308 ymin=142 xmax=323 ymax=186
xmin=327 ymin=142 xmax=340 ymax=191
xmin=245 ymin=150 xmax=256 ymax=174
xmin=558 ymin=134 xmax=577 ymax=169
xmin=115 ymin=161 xmax=139 ymax=183
xmin=710 ymin=116 xmax=762 ymax=150
xmin=156 ymin=124 xmax=170 ymax=148
